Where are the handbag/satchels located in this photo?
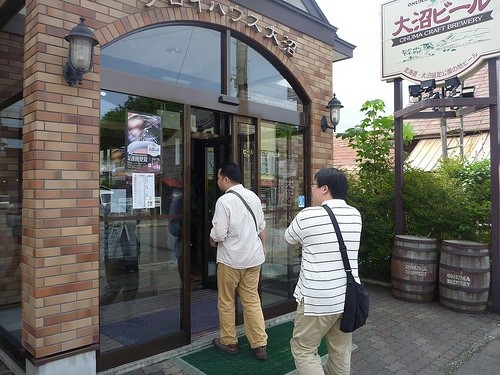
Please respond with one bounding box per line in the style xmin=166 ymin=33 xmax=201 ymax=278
xmin=340 ymin=281 xmax=369 ymax=333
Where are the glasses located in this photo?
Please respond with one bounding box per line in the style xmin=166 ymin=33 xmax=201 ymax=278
xmin=310 ymin=183 xmax=319 ymax=187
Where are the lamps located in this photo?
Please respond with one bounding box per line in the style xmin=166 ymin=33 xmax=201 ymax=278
xmin=63 ymin=15 xmax=99 ymax=86
xmin=408 ymin=77 xmax=476 ymax=98
xmin=320 ymin=93 xmax=345 ymax=133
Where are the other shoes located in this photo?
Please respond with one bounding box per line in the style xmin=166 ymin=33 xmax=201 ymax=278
xmin=212 ymin=338 xmax=238 ymax=353
xmin=254 ymin=347 xmax=267 ymax=359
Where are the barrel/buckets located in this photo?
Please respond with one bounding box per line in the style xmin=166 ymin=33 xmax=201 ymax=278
xmin=390 ymin=234 xmax=440 ymax=305
xmin=438 ymin=239 xmax=491 ymax=314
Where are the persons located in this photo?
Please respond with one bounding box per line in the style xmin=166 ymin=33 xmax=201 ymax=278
xmin=284 ymin=168 xmax=363 ymax=375
xmin=209 ymin=162 xmax=269 ymax=361
xmin=167 ymin=195 xmax=187 ymax=331
xmin=99 ymin=183 xmax=146 ymax=326
